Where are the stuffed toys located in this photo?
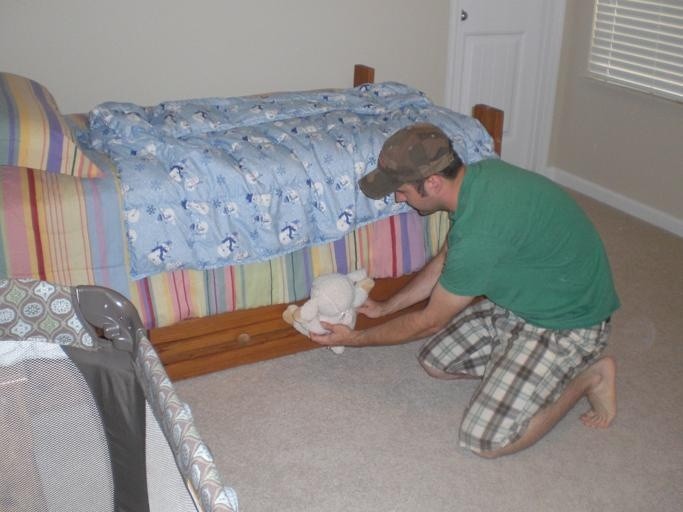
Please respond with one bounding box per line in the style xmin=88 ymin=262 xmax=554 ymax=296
xmin=282 ymin=269 xmax=374 ymax=354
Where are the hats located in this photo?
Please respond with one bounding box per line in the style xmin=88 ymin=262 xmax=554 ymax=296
xmin=358 ymin=123 xmax=455 ymax=200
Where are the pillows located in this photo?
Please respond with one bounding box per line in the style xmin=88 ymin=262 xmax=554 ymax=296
xmin=0 ymin=67 xmax=104 ymax=179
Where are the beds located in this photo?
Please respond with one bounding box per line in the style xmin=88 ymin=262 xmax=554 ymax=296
xmin=0 ymin=61 xmax=506 ymax=383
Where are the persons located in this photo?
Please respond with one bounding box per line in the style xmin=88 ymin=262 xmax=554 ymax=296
xmin=309 ymin=123 xmax=616 ymax=459
